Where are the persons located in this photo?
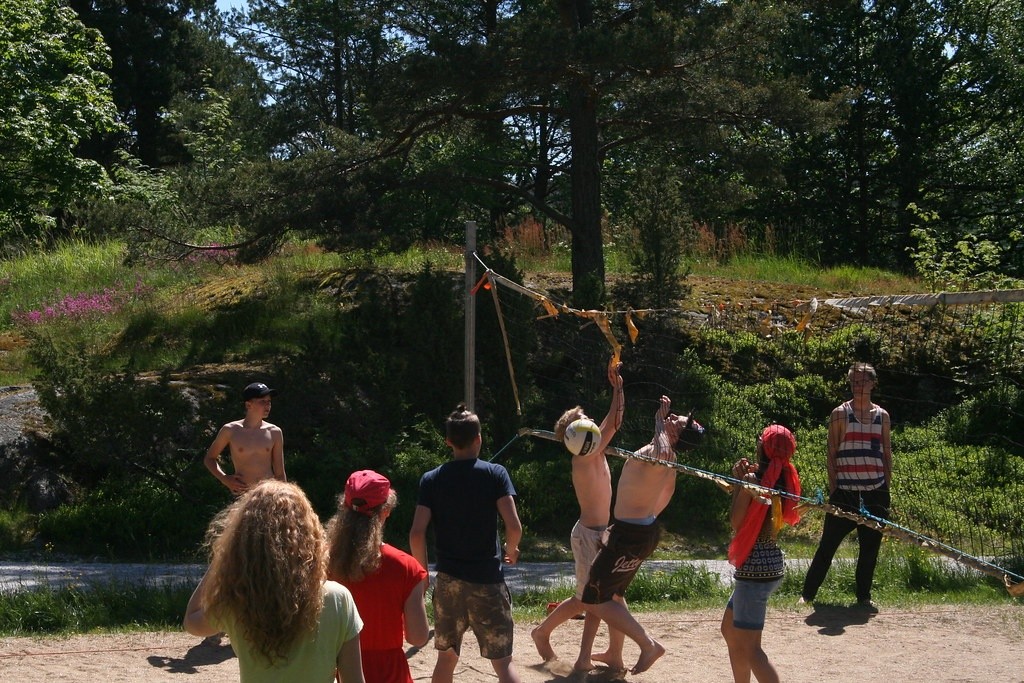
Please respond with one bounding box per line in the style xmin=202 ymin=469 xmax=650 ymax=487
xmin=183 ymin=482 xmax=367 ymax=683
xmin=581 ymin=395 xmax=704 ymax=675
xmin=721 ymin=424 xmax=802 ymax=683
xmin=204 ymin=382 xmax=288 ymax=496
xmin=531 ymin=359 xmax=626 ymax=671
xmin=409 ymin=404 xmax=524 ymax=683
xmin=326 ymin=469 xmax=429 ymax=683
xmin=795 ymin=362 xmax=893 ymax=615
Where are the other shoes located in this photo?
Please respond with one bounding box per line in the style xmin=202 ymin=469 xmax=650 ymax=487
xmin=853 ymin=600 xmax=879 ymax=612
xmin=201 ymin=636 xmax=222 ymax=649
xmin=546 ymin=601 xmax=585 ymax=619
xmin=796 ymin=596 xmax=816 ymax=606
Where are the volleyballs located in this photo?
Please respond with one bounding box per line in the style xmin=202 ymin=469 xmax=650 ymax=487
xmin=563 ymin=419 xmax=602 ymax=457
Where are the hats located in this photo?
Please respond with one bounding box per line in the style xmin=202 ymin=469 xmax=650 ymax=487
xmin=674 ymin=413 xmax=706 ymax=451
xmin=241 ymin=381 xmax=278 ymax=402
xmin=344 ymin=469 xmax=391 ymax=512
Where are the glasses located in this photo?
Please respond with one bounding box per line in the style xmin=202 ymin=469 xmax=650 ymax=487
xmin=847 ymin=379 xmax=872 ymax=385
